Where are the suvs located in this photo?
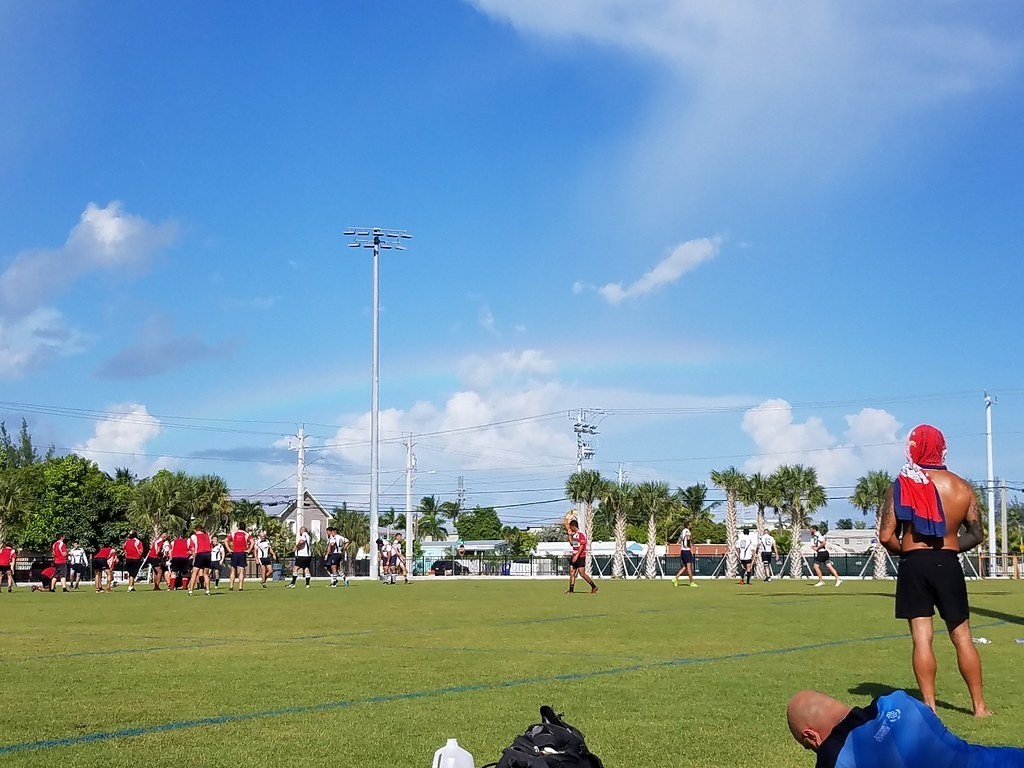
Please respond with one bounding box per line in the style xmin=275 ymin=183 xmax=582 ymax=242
xmin=431 ymin=560 xmax=470 ymax=576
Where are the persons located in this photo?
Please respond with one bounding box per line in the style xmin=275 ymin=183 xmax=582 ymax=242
xmin=880 ymin=424 xmax=994 ymax=717
xmin=254 ymin=532 xmax=275 ymax=589
xmin=32 ymin=566 xmax=56 ymax=592
xmin=148 ymin=531 xmax=204 ymax=591
xmin=93 ymin=545 xmax=119 ymax=593
xmin=68 ymin=541 xmax=89 ymax=589
xmin=285 ymin=526 xmax=312 ymax=588
xmin=376 ymin=533 xmax=414 ymax=586
xmin=756 ymin=529 xmax=779 ymax=582
xmin=810 ymin=526 xmax=843 ymax=587
xmin=209 ymin=535 xmax=225 ymax=588
xmin=672 ymin=521 xmax=699 ymax=587
xmin=50 ymin=533 xmax=69 ymax=592
xmin=565 ymin=520 xmax=599 ymax=594
xmin=123 ymin=530 xmax=144 ymax=593
xmin=188 ymin=525 xmax=212 ymax=596
xmin=787 ymin=689 xmax=1024 ymax=767
xmin=325 ymin=527 xmax=351 ymax=587
xmin=224 ymin=522 xmax=254 ymax=590
xmin=736 ymin=529 xmax=755 ymax=584
xmin=0 ymin=543 xmax=17 ymax=592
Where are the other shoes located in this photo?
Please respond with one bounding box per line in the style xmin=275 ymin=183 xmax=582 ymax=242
xmin=8 ymin=586 xmax=12 ymax=593
xmin=689 ymin=582 xmax=698 ymax=587
xmin=390 ymin=582 xmax=396 ymax=585
xmin=739 ymin=580 xmax=745 ymax=584
xmin=383 ymin=581 xmax=389 ymax=584
xmin=813 ymin=582 xmax=826 ymax=587
xmin=62 ymin=588 xmax=68 ymax=593
xmin=764 ymin=577 xmax=771 ymax=582
xmin=31 ymin=585 xmax=37 ymax=592
xmin=75 ymin=586 xmax=79 ymax=590
xmin=671 ymin=577 xmax=678 ymax=587
xmin=591 ymin=587 xmax=598 ymax=595
xmin=405 ymin=580 xmax=414 ymax=584
xmin=51 ymin=589 xmax=55 ymax=591
xmin=68 ymin=584 xmax=73 ymax=588
xmin=94 ymin=575 xmax=353 ymax=595
xmin=835 ymin=579 xmax=843 ymax=587
xmin=564 ymin=591 xmax=574 ymax=594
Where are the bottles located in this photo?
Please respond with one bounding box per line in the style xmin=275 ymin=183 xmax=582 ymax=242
xmin=432 ymin=738 xmax=475 ymax=768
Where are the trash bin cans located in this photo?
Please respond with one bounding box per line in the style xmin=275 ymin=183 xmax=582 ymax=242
xmin=272 ymin=564 xmax=283 ymax=581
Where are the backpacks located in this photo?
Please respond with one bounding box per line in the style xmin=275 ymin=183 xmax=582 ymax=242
xmin=481 ymin=705 xmax=604 ymax=768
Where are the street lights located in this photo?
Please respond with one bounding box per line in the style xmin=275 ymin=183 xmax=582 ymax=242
xmin=345 ymin=227 xmax=414 ymax=578
